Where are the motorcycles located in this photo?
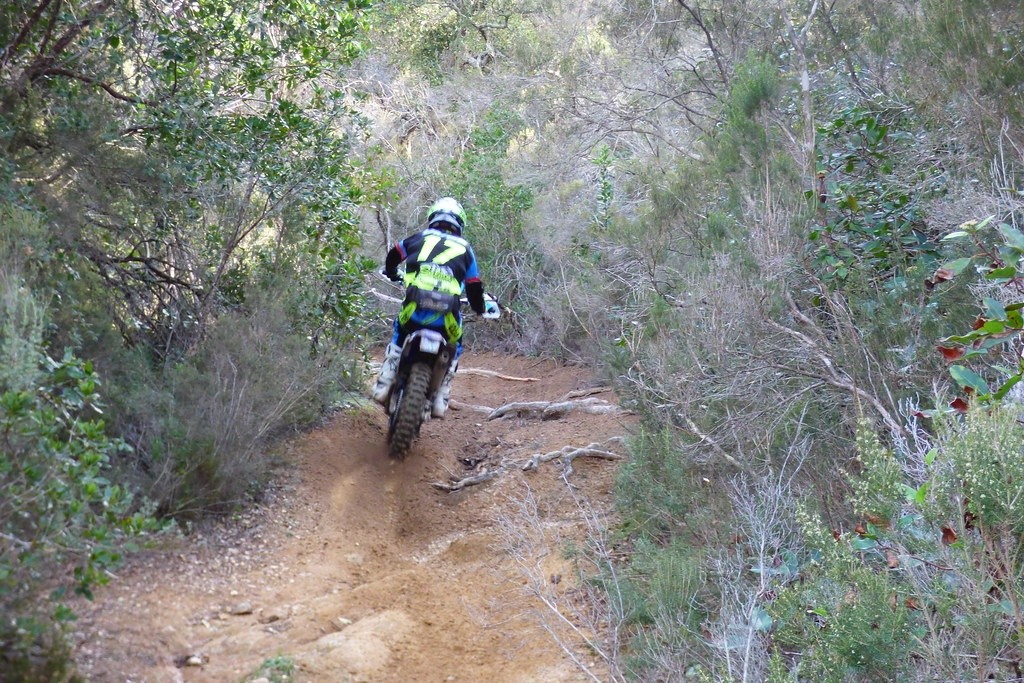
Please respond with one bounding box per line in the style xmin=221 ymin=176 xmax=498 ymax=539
xmin=372 ymin=264 xmax=512 ymax=456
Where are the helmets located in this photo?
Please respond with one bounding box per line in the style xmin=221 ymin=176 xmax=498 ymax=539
xmin=427 ymin=197 xmax=467 ymax=237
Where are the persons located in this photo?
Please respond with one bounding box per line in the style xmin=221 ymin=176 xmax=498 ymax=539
xmin=375 ymin=197 xmax=486 ymax=419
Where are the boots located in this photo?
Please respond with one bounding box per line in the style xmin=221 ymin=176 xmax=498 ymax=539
xmin=374 ymin=342 xmax=401 ymax=400
xmin=431 ymin=357 xmax=457 ymax=420
